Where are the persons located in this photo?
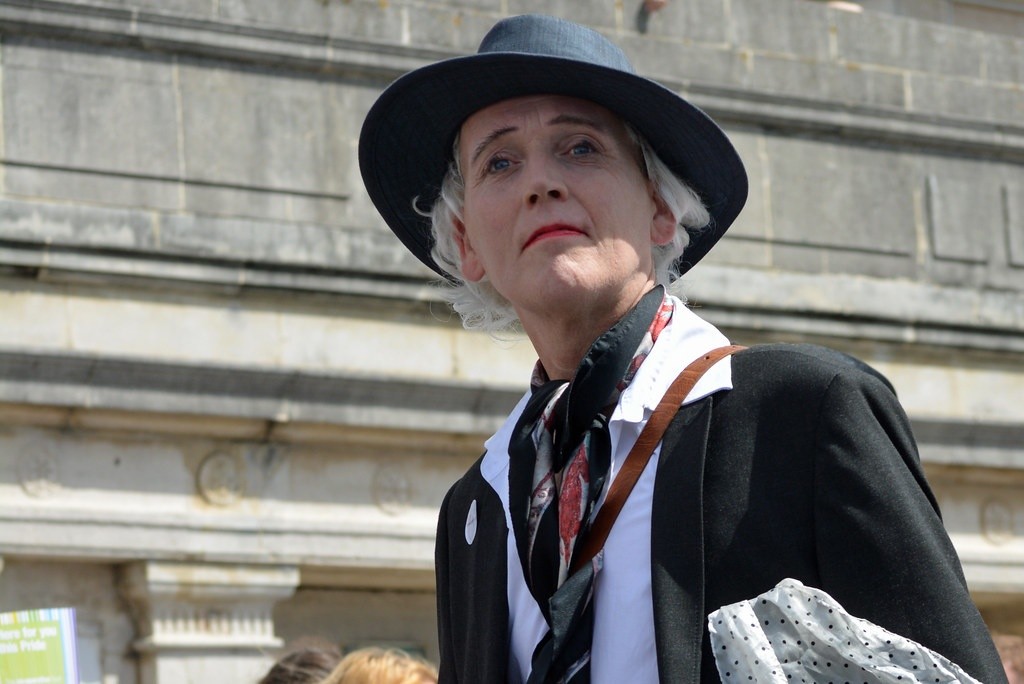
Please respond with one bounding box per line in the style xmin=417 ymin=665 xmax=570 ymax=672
xmin=359 ymin=15 xmax=1010 ymax=684
xmin=258 ymin=646 xmax=437 ymax=684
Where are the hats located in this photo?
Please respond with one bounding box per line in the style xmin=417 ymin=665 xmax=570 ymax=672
xmin=358 ymin=16 xmax=750 ymax=287
